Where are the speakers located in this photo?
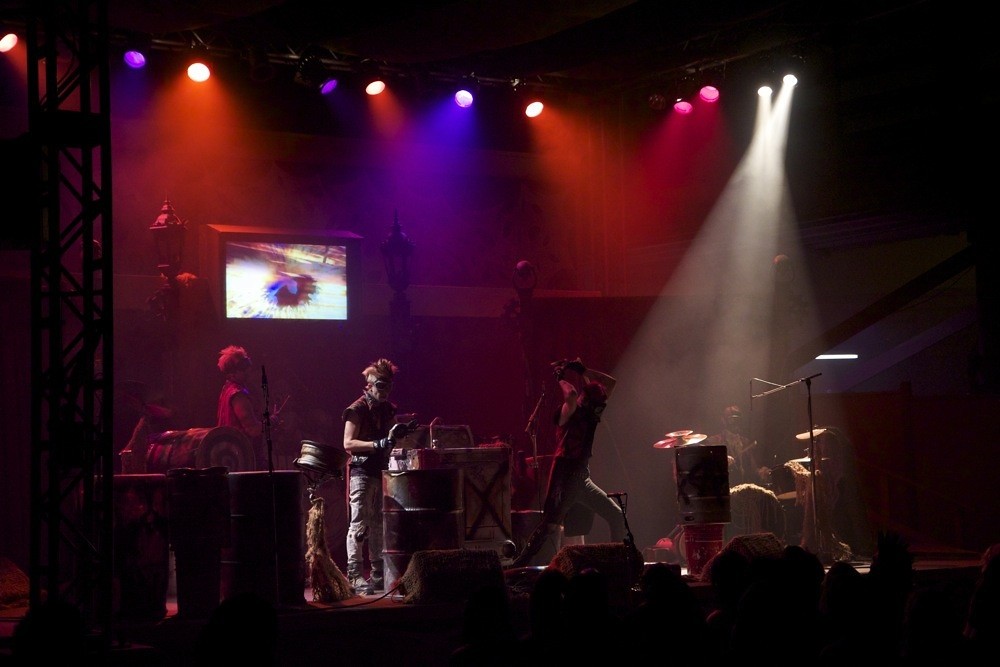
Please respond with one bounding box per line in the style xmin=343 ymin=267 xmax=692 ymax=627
xmin=400 ymin=548 xmax=505 ymax=605
xmin=549 ymin=543 xmax=644 ymax=576
xmin=700 ymin=533 xmax=784 ymax=583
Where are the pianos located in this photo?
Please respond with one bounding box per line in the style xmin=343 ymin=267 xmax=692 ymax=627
xmin=387 ymin=447 xmax=514 ymax=540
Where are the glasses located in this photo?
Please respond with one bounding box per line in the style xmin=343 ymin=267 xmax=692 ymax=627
xmin=373 ymin=381 xmax=397 ymax=391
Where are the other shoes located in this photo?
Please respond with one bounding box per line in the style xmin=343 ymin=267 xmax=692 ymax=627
xmin=370 ymin=569 xmax=384 ymax=590
xmin=347 ymin=571 xmax=374 ymax=595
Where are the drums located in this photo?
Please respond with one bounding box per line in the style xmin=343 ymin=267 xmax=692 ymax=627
xmin=777 ymin=491 xmax=805 ymax=531
xmin=730 ymin=484 xmax=786 ymax=542
xmin=296 ymin=440 xmax=351 ymax=475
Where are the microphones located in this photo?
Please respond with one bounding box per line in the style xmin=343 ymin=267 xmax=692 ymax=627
xmin=749 ymin=380 xmax=753 ymax=411
xmin=607 ymin=492 xmax=626 ymax=498
xmin=261 ymin=365 xmax=268 ymax=385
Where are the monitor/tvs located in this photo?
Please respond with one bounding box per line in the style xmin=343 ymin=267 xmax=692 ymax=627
xmin=220 ymin=239 xmax=350 ymax=323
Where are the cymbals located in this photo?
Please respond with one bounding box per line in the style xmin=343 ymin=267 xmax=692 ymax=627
xmin=653 ymin=433 xmax=707 ymax=449
xmin=795 ymin=429 xmax=825 ymax=440
xmin=790 ymin=456 xmax=830 ymax=463
xmin=665 ymin=430 xmax=693 ymax=437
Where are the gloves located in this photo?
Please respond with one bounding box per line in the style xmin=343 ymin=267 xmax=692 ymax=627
xmin=374 ymin=436 xmax=388 ymax=450
xmin=551 ymin=364 xmax=566 ymax=384
xmin=564 ymin=357 xmax=586 ymax=375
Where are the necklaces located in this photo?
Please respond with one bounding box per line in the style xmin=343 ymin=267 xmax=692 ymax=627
xmin=0 ymin=597 xmax=124 ymax=667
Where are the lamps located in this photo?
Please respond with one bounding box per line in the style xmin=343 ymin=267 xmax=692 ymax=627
xmin=512 ymin=77 xmax=544 ymax=118
xmin=455 ymin=74 xmax=481 ymax=108
xmin=123 ymin=35 xmax=152 ymax=69
xmin=671 ymin=64 xmax=728 ymax=115
xmin=356 ymin=55 xmax=386 ymax=96
xmin=187 ymin=44 xmax=211 ymax=83
xmin=756 ymin=48 xmax=809 ymax=98
xmin=293 ymin=53 xmax=344 ymax=95
xmin=645 ymin=81 xmax=671 ymax=110
xmin=0 ymin=23 xmax=18 ymax=54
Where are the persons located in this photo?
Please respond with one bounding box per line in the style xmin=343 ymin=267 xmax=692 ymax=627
xmin=702 ymin=401 xmax=773 ymax=489
xmin=186 ymin=607 xmax=278 ymax=667
xmin=450 ymin=528 xmax=998 ymax=667
xmin=543 ymin=355 xmax=625 ymax=546
xmin=342 ymin=357 xmax=412 ymax=594
xmin=215 ymin=344 xmax=286 ymax=469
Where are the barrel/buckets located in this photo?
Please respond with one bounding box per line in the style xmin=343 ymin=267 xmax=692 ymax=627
xmin=380 ymin=469 xmax=465 ymax=601
xmin=683 ymin=524 xmax=725 ymax=575
xmin=674 ymin=445 xmax=732 ymax=525
xmin=510 ymin=509 xmax=585 ymax=567
xmin=224 ymin=471 xmax=306 ymax=605
xmin=74 ymin=473 xmax=170 ymax=624
